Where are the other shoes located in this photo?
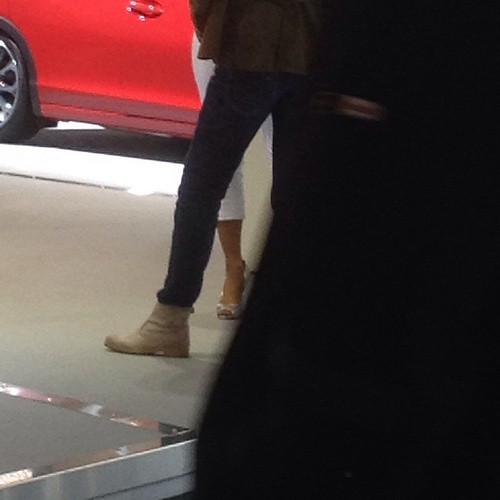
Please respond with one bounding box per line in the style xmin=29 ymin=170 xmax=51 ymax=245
xmin=216 ymin=272 xmax=244 ymax=322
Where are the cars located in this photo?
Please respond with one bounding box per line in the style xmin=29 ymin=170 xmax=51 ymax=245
xmin=0 ymin=2 xmax=209 ymax=146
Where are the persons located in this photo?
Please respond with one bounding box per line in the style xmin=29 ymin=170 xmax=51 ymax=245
xmin=191 ymin=27 xmax=282 ymax=322
xmin=104 ymin=0 xmax=315 ymax=362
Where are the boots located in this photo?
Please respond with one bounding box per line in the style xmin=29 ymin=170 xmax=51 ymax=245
xmin=103 ymin=298 xmax=193 ymax=356
